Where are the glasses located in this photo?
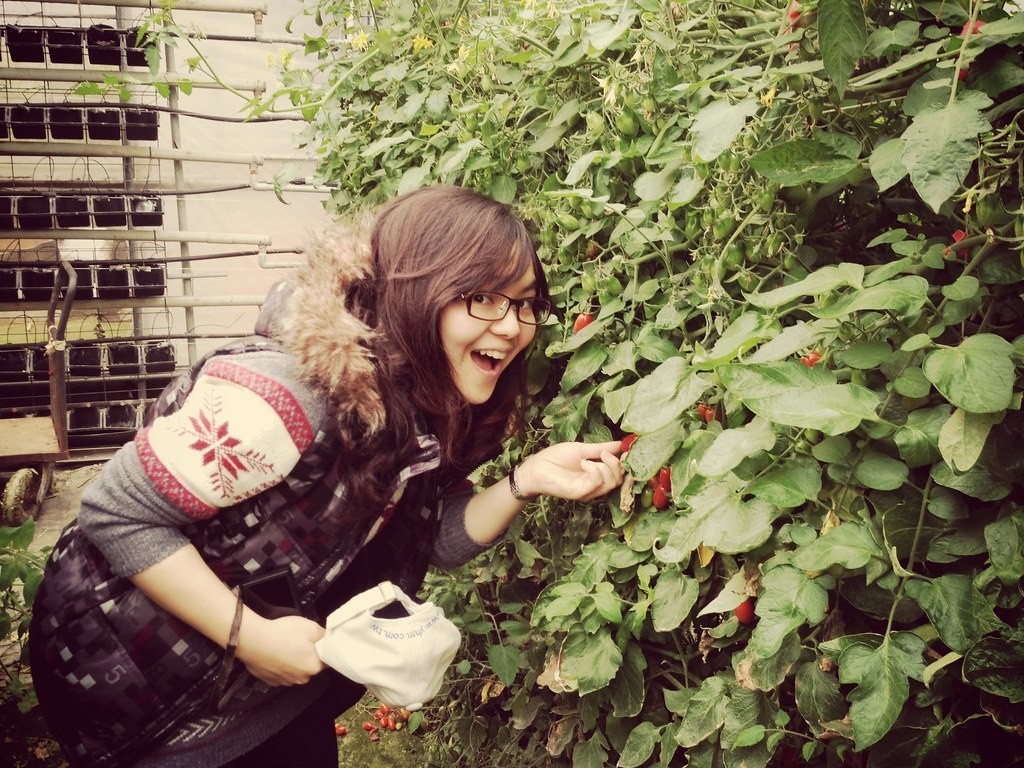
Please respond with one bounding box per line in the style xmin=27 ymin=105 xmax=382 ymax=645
xmin=459 ymin=291 xmax=551 ymax=325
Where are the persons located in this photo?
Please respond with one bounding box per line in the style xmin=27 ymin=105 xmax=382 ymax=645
xmin=29 ymin=184 xmax=624 ymax=768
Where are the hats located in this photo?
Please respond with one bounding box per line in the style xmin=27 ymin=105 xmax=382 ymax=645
xmin=315 ymin=581 xmax=462 ymax=710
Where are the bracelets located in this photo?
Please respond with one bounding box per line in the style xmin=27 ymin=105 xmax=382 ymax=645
xmin=509 ymin=453 xmax=540 ymax=501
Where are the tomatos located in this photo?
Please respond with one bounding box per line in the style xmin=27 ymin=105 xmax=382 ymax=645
xmin=567 ymin=312 xmax=827 ymax=626
xmin=334 ymin=703 xmax=413 ymax=734
